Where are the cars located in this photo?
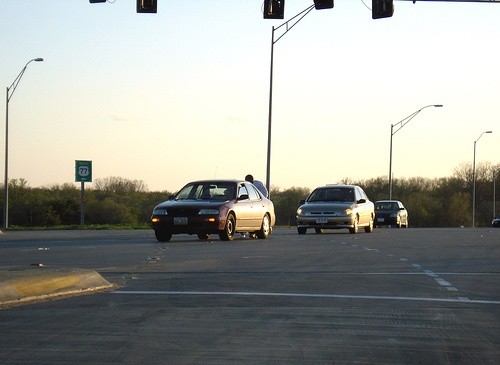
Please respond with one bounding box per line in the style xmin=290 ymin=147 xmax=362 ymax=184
xmin=149 ymin=179 xmax=277 ymax=243
xmin=373 ymin=200 xmax=408 ymax=229
xmin=491 ymin=217 xmax=500 ymax=228
xmin=295 ymin=183 xmax=376 ymax=236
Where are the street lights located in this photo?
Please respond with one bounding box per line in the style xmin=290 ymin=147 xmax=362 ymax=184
xmin=388 ymin=104 xmax=444 ymax=200
xmin=472 ymin=131 xmax=494 ymax=229
xmin=2 ymin=57 xmax=44 ymax=225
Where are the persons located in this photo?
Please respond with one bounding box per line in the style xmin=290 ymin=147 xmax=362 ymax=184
xmin=245 ymin=174 xmax=268 ymax=197
